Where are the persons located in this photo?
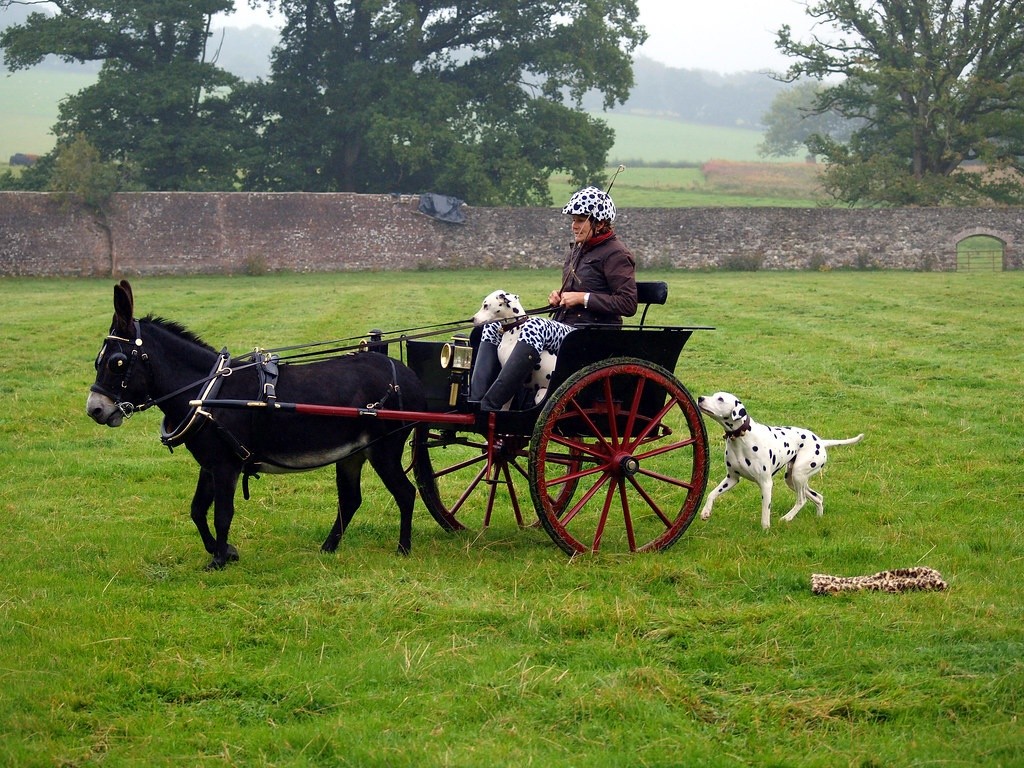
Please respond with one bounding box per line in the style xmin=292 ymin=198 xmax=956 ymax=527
xmin=469 ymin=186 xmax=639 ymax=412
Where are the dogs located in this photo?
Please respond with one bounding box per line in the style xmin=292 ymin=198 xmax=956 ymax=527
xmin=470 ymin=290 xmax=557 ymax=410
xmin=697 ymin=391 xmax=865 ymax=529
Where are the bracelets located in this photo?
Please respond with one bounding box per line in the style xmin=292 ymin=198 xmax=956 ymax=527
xmin=583 ymin=293 xmax=590 ymax=308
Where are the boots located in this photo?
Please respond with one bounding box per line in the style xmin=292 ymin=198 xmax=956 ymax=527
xmin=481 ymin=341 xmax=542 ymax=410
xmin=470 ymin=341 xmax=498 ymax=401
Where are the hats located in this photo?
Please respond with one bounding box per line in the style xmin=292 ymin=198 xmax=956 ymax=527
xmin=561 ymin=186 xmax=617 ymax=223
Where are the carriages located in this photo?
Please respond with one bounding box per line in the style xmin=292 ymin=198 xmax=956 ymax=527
xmin=85 ymin=279 xmax=717 ymax=570
